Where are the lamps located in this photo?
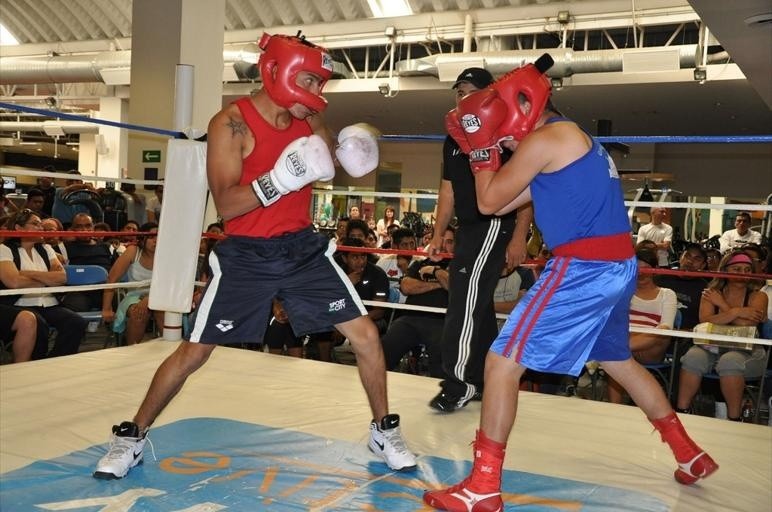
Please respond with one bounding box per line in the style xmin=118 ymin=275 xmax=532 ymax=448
xmin=693 ymin=66 xmax=707 ymax=84
xmin=385 ymin=25 xmax=396 ymax=38
xmin=378 ymin=82 xmax=390 ymax=94
xmin=557 ymin=11 xmax=570 ymax=24
xmin=45 ymin=97 xmax=56 ymax=107
xmin=551 ymin=77 xmax=563 ymax=88
xmin=47 ymin=50 xmax=60 ymax=57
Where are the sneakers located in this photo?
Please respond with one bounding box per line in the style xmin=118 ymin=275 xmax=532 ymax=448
xmin=91 ymin=421 xmax=156 ymax=480
xmin=428 ymin=389 xmax=476 ymax=412
xmin=368 ymin=413 xmax=419 ymax=472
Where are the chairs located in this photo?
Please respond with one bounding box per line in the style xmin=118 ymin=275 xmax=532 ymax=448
xmin=0 ymin=189 xmax=158 ymax=364
xmin=386 ymin=287 xmax=399 ymax=335
xmin=527 ymin=310 xmax=772 ymax=424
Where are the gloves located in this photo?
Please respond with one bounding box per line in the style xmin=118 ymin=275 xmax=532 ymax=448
xmin=443 ymin=110 xmax=471 ymax=159
xmin=457 ymin=86 xmax=508 ymax=172
xmin=336 ymin=123 xmax=380 ymax=179
xmin=251 ymin=136 xmax=335 ymax=207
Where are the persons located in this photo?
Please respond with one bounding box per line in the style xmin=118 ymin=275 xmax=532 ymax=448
xmin=428 ymin=69 xmax=534 ymax=414
xmin=2 ymin=171 xmax=164 ymax=364
xmin=587 ymin=207 xmax=772 ymax=423
xmin=423 ymin=55 xmax=719 ymax=511
xmin=93 ymin=29 xmax=416 ymax=480
xmin=181 ymin=206 xmax=461 ymax=377
xmin=494 ymin=226 xmax=550 ymax=314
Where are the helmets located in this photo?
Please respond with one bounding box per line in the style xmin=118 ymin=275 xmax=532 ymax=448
xmin=257 ymin=31 xmax=333 ymax=115
xmin=485 ymin=52 xmax=554 ymax=145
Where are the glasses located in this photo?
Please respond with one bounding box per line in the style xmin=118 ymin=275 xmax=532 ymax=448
xmin=17 ymin=208 xmax=34 ymax=226
xmin=707 ymin=255 xmax=719 ymax=260
xmin=752 ymin=257 xmax=762 ymax=263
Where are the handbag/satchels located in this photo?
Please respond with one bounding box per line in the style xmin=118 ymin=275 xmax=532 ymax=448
xmin=693 ymin=323 xmax=760 ymax=353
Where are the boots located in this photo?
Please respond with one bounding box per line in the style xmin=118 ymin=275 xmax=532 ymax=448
xmin=646 ymin=410 xmax=719 ymax=485
xmin=424 ymin=426 xmax=506 ymax=511
